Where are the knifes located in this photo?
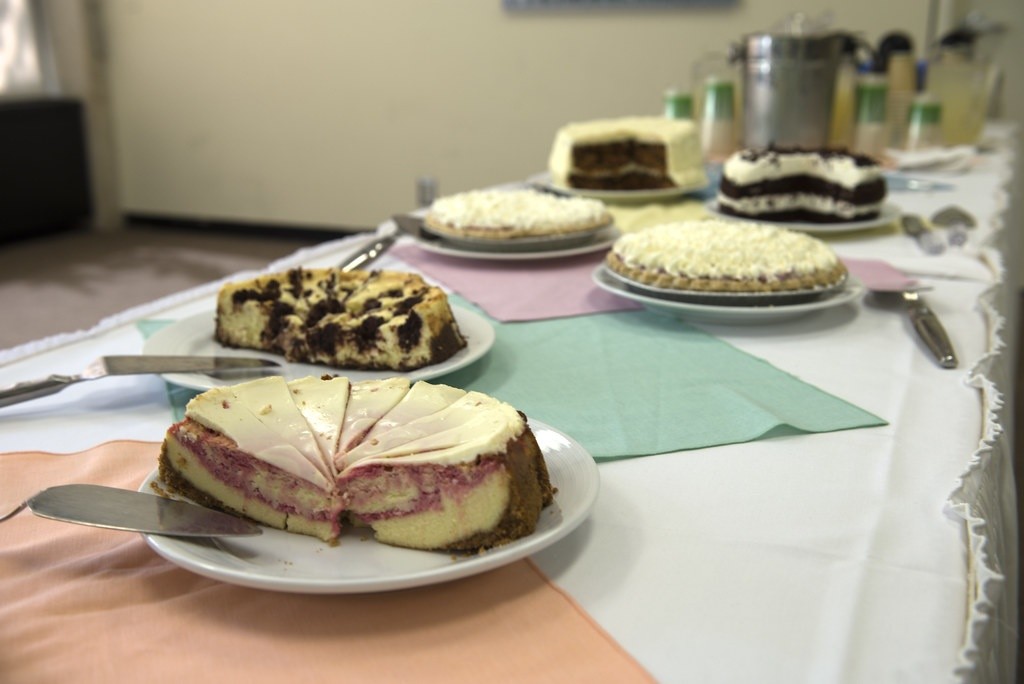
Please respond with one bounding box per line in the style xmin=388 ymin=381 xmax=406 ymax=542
xmin=2 ymin=485 xmax=262 ymax=536
xmin=870 ymin=278 xmax=959 ymax=367
xmin=1 ymin=354 xmax=280 ymax=407
xmin=903 ymin=208 xmax=974 ymax=254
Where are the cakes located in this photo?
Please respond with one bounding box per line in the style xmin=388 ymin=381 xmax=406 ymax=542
xmin=549 ymin=116 xmax=709 ymax=190
xmin=606 ymin=219 xmax=842 ymax=292
xmin=425 ymin=188 xmax=611 ymax=236
xmin=717 ymin=146 xmax=888 ymax=222
xmin=157 ymin=375 xmax=554 ymax=550
xmin=216 ymin=269 xmax=466 ymax=372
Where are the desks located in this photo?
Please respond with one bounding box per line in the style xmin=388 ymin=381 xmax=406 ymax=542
xmin=0 ymin=124 xmax=1024 ymax=684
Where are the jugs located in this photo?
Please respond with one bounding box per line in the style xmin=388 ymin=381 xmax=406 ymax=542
xmin=727 ymin=12 xmax=880 ymax=148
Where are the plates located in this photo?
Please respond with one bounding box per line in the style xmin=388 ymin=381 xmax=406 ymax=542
xmin=593 ymin=266 xmax=862 ymax=324
xmin=138 ymin=417 xmax=600 ymax=593
xmin=146 ymin=309 xmax=496 ymax=391
xmin=535 ymin=169 xmax=707 ymax=206
xmin=710 ymin=202 xmax=895 ymax=237
xmin=412 ymin=207 xmax=618 ymax=260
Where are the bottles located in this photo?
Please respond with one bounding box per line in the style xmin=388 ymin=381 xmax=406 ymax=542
xmin=663 ymin=76 xmax=735 ymax=155
xmin=826 ymin=23 xmax=1008 ymax=150
xmin=0 ymin=2 xmax=93 ymax=243
